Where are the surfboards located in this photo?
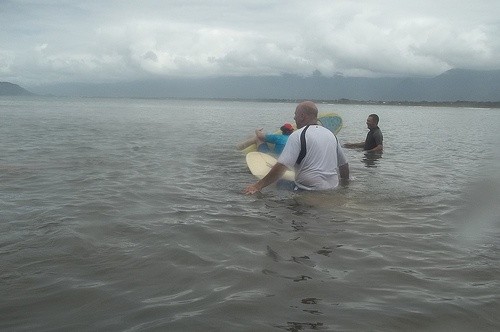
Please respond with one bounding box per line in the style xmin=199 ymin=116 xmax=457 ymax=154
xmin=246 ymin=152 xmax=295 ymax=189
xmin=232 ymin=112 xmax=343 ymax=154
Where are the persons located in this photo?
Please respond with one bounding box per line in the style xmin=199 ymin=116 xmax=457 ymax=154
xmin=243 ymin=101 xmax=349 ymax=196
xmin=343 ymin=114 xmax=383 ymax=151
xmin=235 ymin=124 xmax=294 ymax=155
